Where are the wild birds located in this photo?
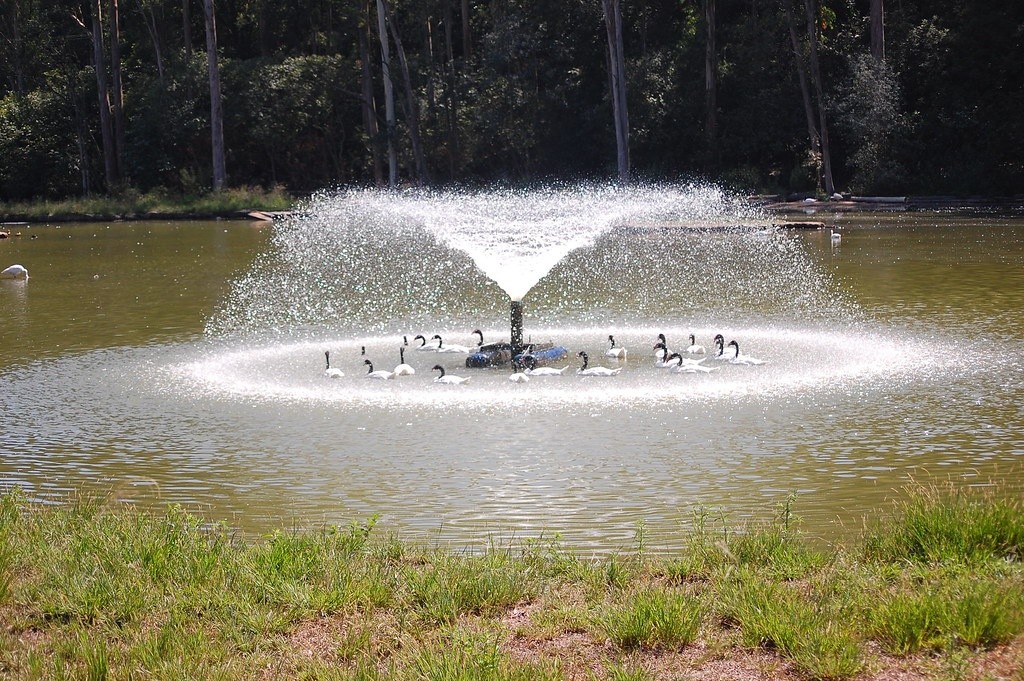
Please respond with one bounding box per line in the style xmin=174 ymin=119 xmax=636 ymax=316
xmin=830 ymin=193 xmax=843 ymax=203
xmin=403 ymin=329 xmax=511 ymax=357
xmin=361 ymin=346 xmax=395 ymax=381
xmin=803 ymin=195 xmax=818 ymax=203
xmin=394 ymin=347 xmax=415 ymax=376
xmin=324 ymin=350 xmax=345 ymax=379
xmin=830 ymin=229 xmax=841 ymax=238
xmin=431 ymin=364 xmax=471 ymax=386
xmin=0 ymin=264 xmax=29 ymax=280
xmin=606 ymin=334 xmax=628 ymax=362
xmin=508 ymin=355 xmax=570 ymax=384
xmin=651 ymin=333 xmax=715 ymax=374
xmin=575 ymin=352 xmax=622 ymax=378
xmin=713 ymin=333 xmax=770 ymax=367
